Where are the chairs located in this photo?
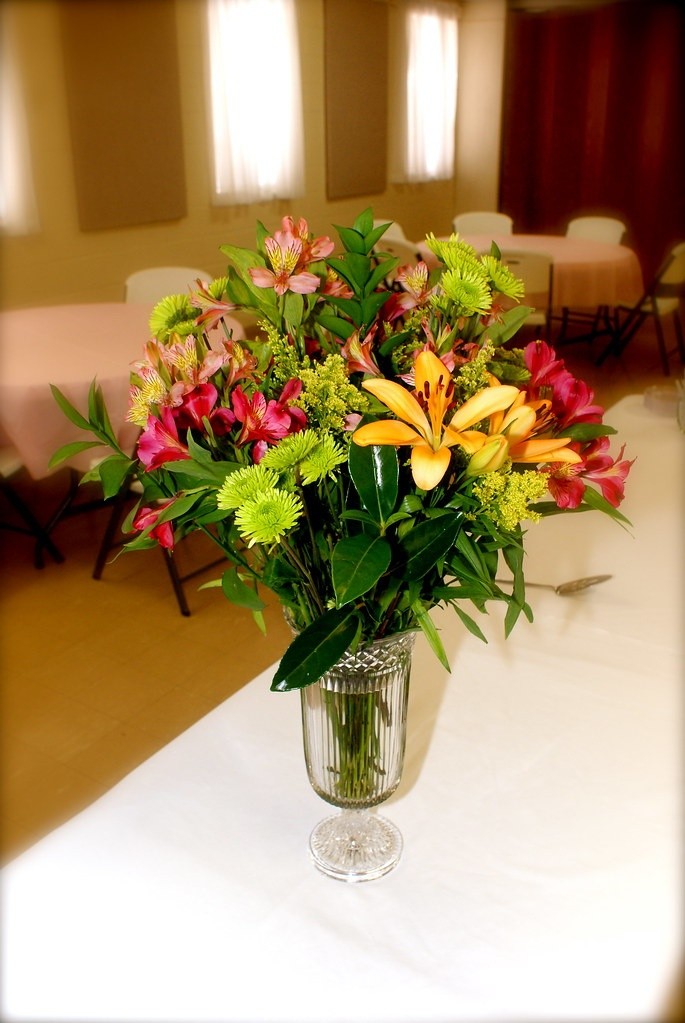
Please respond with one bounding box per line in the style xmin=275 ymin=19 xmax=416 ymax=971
xmin=597 ymin=237 xmax=685 ymax=379
xmin=562 ymin=214 xmax=624 ymax=344
xmin=0 ymin=427 xmax=72 ymax=573
xmin=125 ymin=256 xmax=218 ymax=309
xmin=474 ymin=251 xmax=553 ymax=343
xmin=370 ymin=224 xmax=426 ymax=326
xmin=454 ymin=209 xmax=513 ymax=238
xmin=367 ymin=216 xmax=413 ymax=292
xmin=63 ymin=393 xmax=245 ymax=621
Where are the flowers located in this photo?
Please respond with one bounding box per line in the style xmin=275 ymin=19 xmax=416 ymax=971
xmin=44 ymin=203 xmax=645 ymax=697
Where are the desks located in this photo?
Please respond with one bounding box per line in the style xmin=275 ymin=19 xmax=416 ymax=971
xmin=0 ymin=301 xmax=246 ymax=576
xmin=420 ymin=235 xmax=642 ymax=364
xmin=0 ymin=391 xmax=685 ymax=1023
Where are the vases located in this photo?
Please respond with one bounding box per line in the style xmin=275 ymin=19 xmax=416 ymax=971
xmin=277 ymin=586 xmax=437 ymax=890
